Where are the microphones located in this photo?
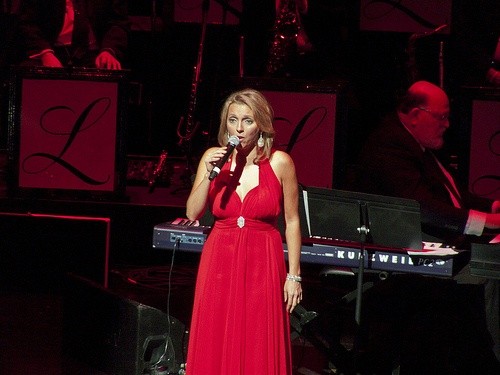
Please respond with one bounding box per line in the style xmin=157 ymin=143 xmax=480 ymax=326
xmin=208 ymin=135 xmax=239 ymax=181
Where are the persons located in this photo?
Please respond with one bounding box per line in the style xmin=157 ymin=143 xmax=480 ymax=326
xmin=239 ymin=0 xmax=336 ymax=86
xmin=0 ymin=0 xmax=128 ymax=70
xmin=184 ymin=89 xmax=303 ymax=375
xmin=358 ymin=78 xmax=500 ymax=241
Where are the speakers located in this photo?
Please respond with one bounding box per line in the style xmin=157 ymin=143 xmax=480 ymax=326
xmin=469 ymin=100 xmax=500 ymax=202
xmin=62 ymin=272 xmax=183 ymax=375
xmin=235 ymin=87 xmax=336 ymax=189
xmin=20 ymin=80 xmax=119 ymax=189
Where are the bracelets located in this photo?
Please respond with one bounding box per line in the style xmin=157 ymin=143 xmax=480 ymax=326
xmin=287 ymin=272 xmax=302 ymax=282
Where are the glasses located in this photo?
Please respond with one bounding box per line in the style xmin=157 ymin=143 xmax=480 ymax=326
xmin=419 ymin=108 xmax=449 ymax=119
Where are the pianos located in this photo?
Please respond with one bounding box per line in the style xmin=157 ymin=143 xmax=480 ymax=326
xmin=150 ymin=186 xmax=467 ymax=375
